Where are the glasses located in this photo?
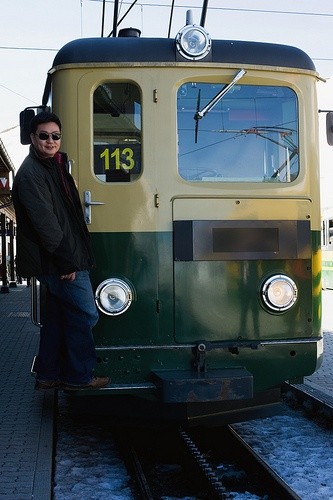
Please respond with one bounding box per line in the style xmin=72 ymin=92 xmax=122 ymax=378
xmin=36 ymin=132 xmax=62 ymax=140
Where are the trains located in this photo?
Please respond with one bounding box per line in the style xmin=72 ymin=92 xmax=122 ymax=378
xmin=16 ymin=0 xmax=333 ymax=425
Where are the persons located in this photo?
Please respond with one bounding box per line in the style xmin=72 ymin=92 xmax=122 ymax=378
xmin=13 ymin=255 xmax=31 ymax=288
xmin=11 ymin=111 xmax=110 ymax=393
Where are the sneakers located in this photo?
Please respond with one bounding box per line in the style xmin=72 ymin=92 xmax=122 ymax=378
xmin=32 ymin=377 xmax=62 ymax=392
xmin=63 ymin=375 xmax=112 ymax=390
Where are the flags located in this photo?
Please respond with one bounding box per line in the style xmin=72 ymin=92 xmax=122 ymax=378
xmin=0 ymin=177 xmax=9 ymax=190
